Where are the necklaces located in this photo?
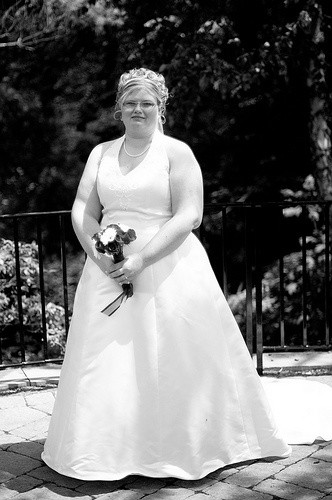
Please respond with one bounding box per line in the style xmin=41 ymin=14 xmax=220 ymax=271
xmin=122 ymin=138 xmax=152 ymax=157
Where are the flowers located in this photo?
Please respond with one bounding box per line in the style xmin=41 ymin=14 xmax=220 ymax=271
xmin=91 ymin=224 xmax=137 ymax=316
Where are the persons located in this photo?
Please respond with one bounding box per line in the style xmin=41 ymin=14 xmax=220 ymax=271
xmin=41 ymin=69 xmax=291 ymax=481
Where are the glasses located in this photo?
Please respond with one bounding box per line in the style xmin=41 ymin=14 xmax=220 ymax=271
xmin=123 ymin=100 xmax=159 ymax=109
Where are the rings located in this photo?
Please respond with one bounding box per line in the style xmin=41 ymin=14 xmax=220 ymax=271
xmin=124 ymin=274 xmax=128 ymax=280
